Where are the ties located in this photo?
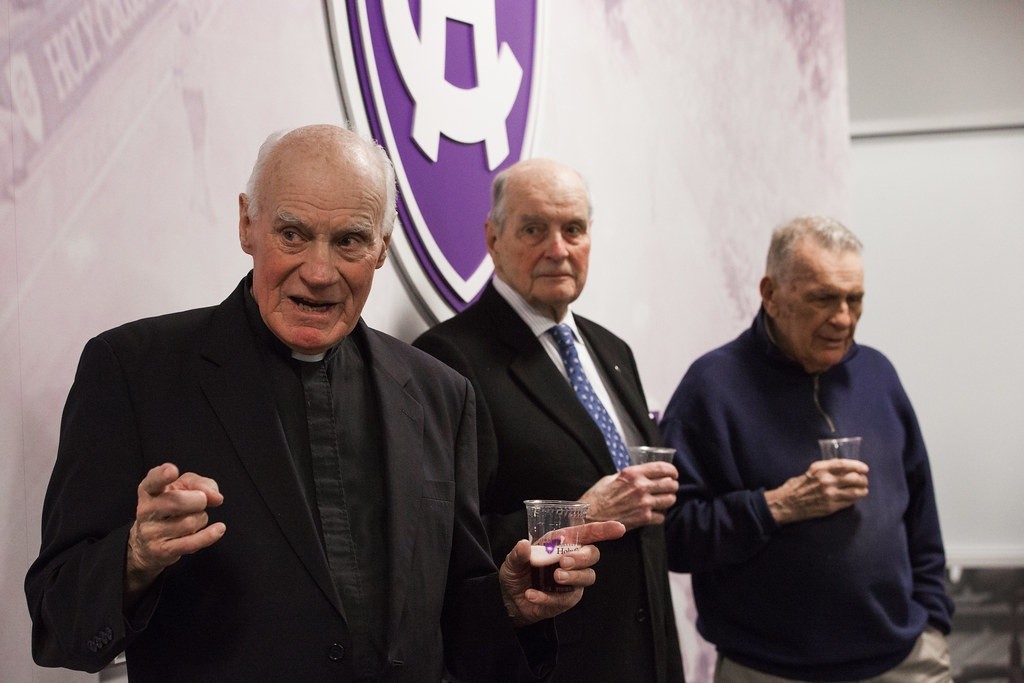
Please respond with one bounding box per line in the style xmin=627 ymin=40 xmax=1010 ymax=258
xmin=547 ymin=322 xmax=632 ymax=470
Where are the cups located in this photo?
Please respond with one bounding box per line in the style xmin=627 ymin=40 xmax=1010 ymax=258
xmin=521 ymin=497 xmax=588 ymax=593
xmin=628 ymin=445 xmax=675 ymax=470
xmin=818 ymin=437 xmax=862 ymax=462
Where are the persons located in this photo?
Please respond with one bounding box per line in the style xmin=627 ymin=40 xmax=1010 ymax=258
xmin=659 ymin=217 xmax=954 ymax=683
xmin=411 ymin=163 xmax=690 ymax=683
xmin=25 ymin=128 xmax=627 ymax=683
